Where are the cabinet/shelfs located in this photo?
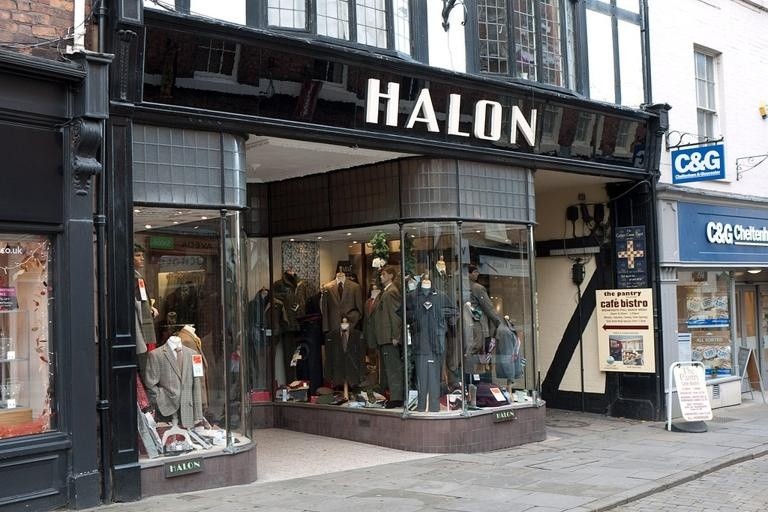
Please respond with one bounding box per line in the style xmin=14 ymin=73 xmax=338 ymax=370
xmin=0 ymin=308 xmax=32 ymax=410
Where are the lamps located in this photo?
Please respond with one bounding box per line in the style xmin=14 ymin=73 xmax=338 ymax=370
xmin=747 ymin=269 xmax=762 ymax=274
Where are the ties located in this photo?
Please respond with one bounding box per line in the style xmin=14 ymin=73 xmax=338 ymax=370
xmin=176 ymin=346 xmax=183 ymax=373
xmin=341 ymin=328 xmax=347 ymax=353
xmin=338 ymin=281 xmax=344 ymax=300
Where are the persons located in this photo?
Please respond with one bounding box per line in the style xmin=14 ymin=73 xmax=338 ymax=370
xmin=406 ymin=276 xmax=454 ymax=411
xmin=322 ymin=268 xmax=364 ymax=333
xmin=468 ymin=266 xmax=501 ymax=357
xmin=270 ymin=268 xmax=306 ymax=383
xmin=363 ymin=283 xmax=382 ymax=316
xmin=177 ymin=323 xmax=210 ymax=411
xmin=246 ymin=288 xmax=288 ymax=403
xmin=135 ymin=244 xmax=159 ymax=380
xmin=428 ymin=255 xmax=462 ymax=391
xmin=366 ymin=267 xmax=404 ymax=409
xmin=144 ymin=336 xmax=201 ymax=450
xmin=324 ymin=317 xmax=368 ymax=402
xmin=495 ymin=315 xmax=527 ymax=405
